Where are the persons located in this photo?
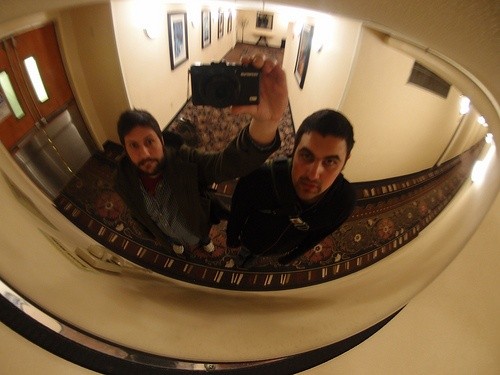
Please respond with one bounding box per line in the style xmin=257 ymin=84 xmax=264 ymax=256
xmin=222 ymin=109 xmax=357 ymax=271
xmin=112 ymin=53 xmax=288 ymax=255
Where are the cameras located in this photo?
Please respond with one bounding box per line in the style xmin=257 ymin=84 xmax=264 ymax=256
xmin=190 ymin=59 xmax=261 ymax=108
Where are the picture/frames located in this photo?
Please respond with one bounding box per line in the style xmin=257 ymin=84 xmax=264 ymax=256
xmin=218 ymin=8 xmax=224 ymax=40
xmin=227 ymin=9 xmax=232 ymax=34
xmin=293 ymin=23 xmax=314 ymax=90
xmin=201 ymin=10 xmax=212 ymax=49
xmin=167 ymin=12 xmax=189 ymax=71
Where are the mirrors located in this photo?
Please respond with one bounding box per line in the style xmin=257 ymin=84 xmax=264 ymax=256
xmin=0 ymin=0 xmax=500 ymax=375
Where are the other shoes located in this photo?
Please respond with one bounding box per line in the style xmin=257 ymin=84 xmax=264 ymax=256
xmin=236 ymin=245 xmax=254 ymax=270
xmin=173 ymin=243 xmax=185 ymax=255
xmin=202 ymin=240 xmax=214 ymax=253
